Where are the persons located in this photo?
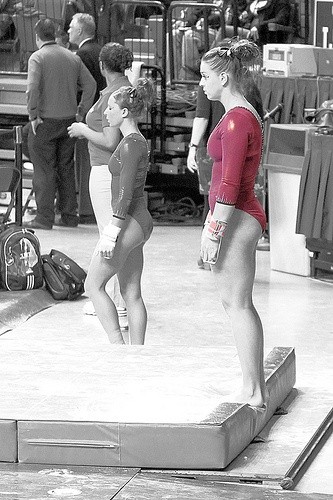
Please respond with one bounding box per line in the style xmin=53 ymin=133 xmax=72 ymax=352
xmin=53 ymin=13 xmax=107 ymax=225
xmin=24 ymin=16 xmax=96 ymax=229
xmin=195 ymin=39 xmax=271 ymax=407
xmin=187 ymin=35 xmax=263 ymax=270
xmin=67 ymin=42 xmax=134 ymax=329
xmin=83 ymin=77 xmax=157 ymax=346
xmin=0 ymin=0 xmax=299 ymax=76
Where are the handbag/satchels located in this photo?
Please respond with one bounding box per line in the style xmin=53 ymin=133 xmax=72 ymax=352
xmin=42 ymin=250 xmax=87 ymax=301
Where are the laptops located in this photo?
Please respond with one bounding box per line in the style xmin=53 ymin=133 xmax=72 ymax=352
xmin=313 ymin=48 xmax=333 ymax=76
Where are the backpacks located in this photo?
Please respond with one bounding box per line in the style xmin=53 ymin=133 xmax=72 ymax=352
xmin=0 ymin=225 xmax=44 ymax=291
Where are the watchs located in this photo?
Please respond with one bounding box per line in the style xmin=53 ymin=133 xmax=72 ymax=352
xmin=189 ymin=143 xmax=198 ymax=148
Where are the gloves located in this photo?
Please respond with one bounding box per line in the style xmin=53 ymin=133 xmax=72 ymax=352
xmin=199 ymin=215 xmax=228 ymax=264
xmin=96 ymin=225 xmax=121 ymax=260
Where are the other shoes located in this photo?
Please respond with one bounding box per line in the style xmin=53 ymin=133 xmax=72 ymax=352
xmin=24 ymin=218 xmax=52 ymax=229
xmin=54 ymin=218 xmax=77 ymax=227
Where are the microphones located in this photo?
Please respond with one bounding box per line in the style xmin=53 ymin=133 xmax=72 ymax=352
xmin=264 ymin=103 xmax=285 ymax=121
